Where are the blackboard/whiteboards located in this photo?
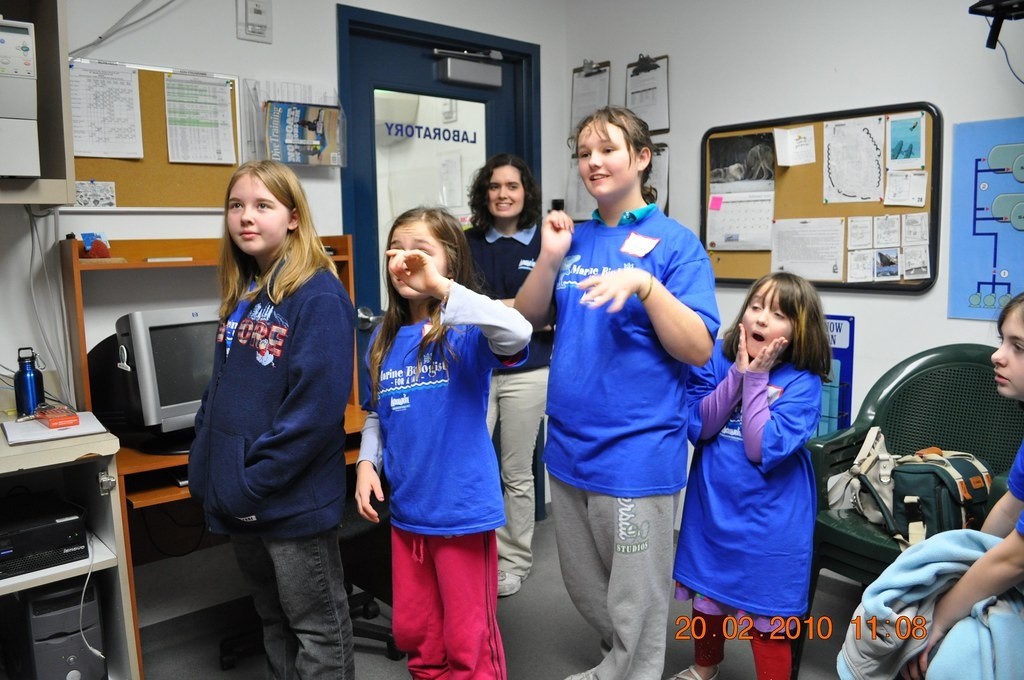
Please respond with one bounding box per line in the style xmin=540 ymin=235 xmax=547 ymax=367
xmin=49 ymin=59 xmax=245 ymax=215
xmin=700 ymin=101 xmax=944 ymax=296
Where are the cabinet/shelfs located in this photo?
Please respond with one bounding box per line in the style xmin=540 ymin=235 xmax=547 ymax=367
xmin=61 ymin=235 xmax=369 ymax=680
xmin=0 ymin=369 xmax=140 ymax=680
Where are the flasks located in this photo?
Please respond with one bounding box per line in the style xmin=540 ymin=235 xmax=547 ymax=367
xmin=14 ymin=347 xmax=44 ymax=419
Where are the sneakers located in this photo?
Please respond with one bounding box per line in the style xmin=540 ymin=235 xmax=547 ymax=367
xmin=494 ymin=570 xmax=528 ymax=596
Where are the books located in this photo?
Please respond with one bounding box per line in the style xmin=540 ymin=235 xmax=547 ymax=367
xmin=2 ymin=411 xmax=107 ymax=447
xmin=265 ymin=100 xmax=342 ymax=166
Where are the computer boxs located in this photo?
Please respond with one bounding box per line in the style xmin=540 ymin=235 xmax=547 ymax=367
xmin=5 ymin=575 xmax=109 ymax=680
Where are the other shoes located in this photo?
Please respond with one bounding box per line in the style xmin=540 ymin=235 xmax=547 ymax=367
xmin=561 ymin=666 xmax=600 ymax=680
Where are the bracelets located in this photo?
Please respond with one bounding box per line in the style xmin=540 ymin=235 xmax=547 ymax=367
xmin=440 ymin=277 xmax=454 ymax=305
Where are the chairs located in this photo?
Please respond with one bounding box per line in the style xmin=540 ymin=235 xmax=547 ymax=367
xmin=219 ymin=485 xmax=406 ymax=670
xmin=792 ymin=343 xmax=1024 ymax=680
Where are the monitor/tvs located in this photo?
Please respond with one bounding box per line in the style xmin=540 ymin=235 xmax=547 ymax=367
xmin=114 ymin=304 xmax=224 ymax=457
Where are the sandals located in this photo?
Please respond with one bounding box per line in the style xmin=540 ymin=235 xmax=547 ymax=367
xmin=668 ymin=664 xmax=719 ymax=680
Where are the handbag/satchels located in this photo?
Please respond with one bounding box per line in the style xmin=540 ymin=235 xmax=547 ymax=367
xmin=827 ymin=425 xmax=901 ymax=525
xmin=890 ymin=446 xmax=995 ymax=551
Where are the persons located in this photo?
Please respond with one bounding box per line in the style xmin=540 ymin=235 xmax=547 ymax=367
xmin=514 ymin=106 xmax=720 ymax=680
xmin=668 ymin=273 xmax=831 ymax=680
xmin=188 ymin=160 xmax=359 ymax=680
xmin=463 ymin=154 xmax=555 ymax=596
xmin=900 ymin=291 xmax=1024 ymax=680
xmin=355 ymin=206 xmax=533 ymax=680
